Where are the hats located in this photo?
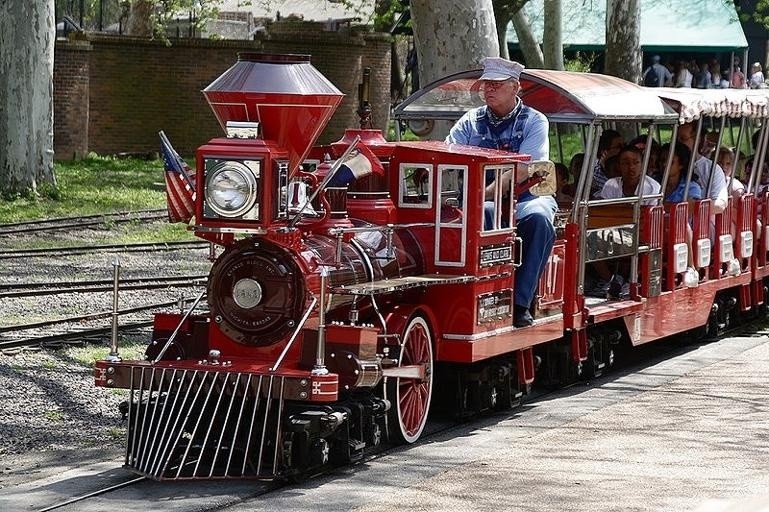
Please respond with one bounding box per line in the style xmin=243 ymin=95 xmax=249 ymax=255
xmin=478 ymin=55 xmax=525 ymax=82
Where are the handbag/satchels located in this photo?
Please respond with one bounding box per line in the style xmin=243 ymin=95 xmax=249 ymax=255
xmin=645 ymin=66 xmax=659 ymax=87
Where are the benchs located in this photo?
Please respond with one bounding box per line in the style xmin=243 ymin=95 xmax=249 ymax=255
xmin=557 ymin=195 xmax=695 ymax=298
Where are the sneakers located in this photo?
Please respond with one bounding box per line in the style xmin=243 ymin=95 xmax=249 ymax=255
xmin=727 ymin=259 xmax=743 ymax=276
xmin=603 ymin=274 xmax=630 ymax=295
xmin=514 ymin=306 xmax=534 ymax=327
xmin=682 ymin=268 xmax=699 ymax=288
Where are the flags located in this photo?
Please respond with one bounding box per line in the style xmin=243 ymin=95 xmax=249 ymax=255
xmin=323 ymin=143 xmax=385 ymax=191
xmin=159 ymin=139 xmax=197 ymax=226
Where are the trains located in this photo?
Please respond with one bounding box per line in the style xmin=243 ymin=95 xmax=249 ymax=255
xmin=92 ymin=48 xmax=768 ymax=495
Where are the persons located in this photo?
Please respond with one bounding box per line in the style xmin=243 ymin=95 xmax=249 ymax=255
xmin=641 ymin=54 xmax=766 ymax=90
xmin=552 ymin=120 xmax=769 ymax=302
xmin=413 ymin=56 xmax=560 ymax=330
